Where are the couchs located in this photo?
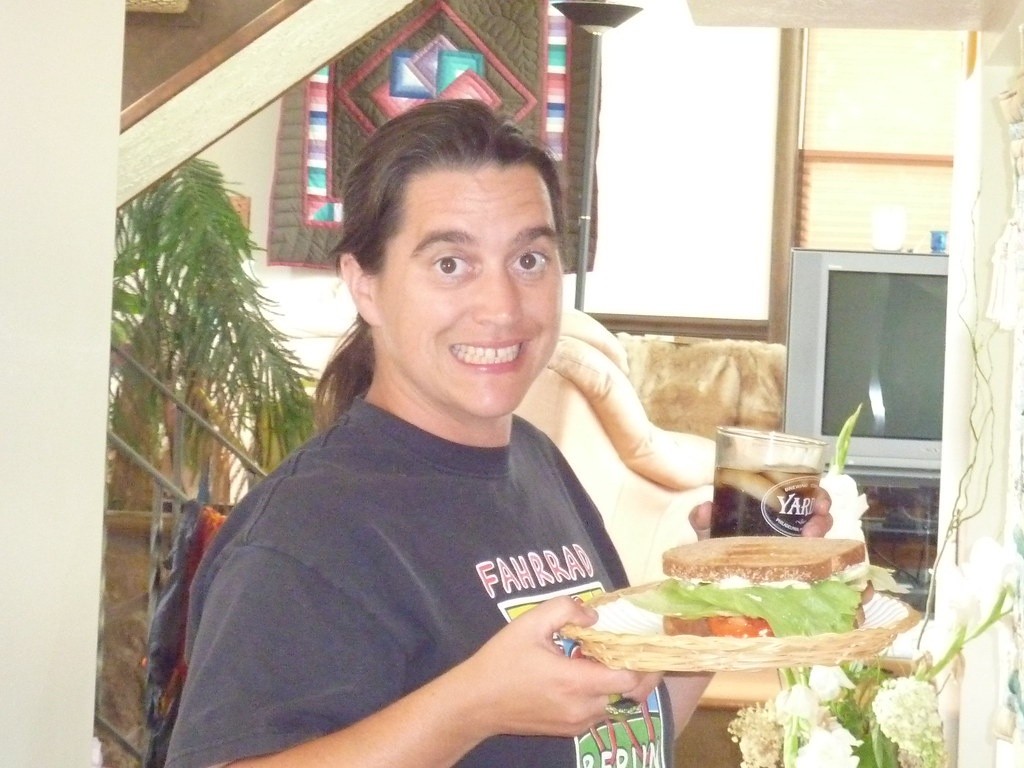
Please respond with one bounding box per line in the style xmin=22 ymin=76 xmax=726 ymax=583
xmin=513 ymin=306 xmax=715 ymax=585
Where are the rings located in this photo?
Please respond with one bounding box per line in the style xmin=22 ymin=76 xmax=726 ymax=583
xmin=605 ymin=695 xmax=642 ymax=724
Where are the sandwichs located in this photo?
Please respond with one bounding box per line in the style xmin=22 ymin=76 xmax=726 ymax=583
xmin=625 ymin=535 xmax=873 ymax=636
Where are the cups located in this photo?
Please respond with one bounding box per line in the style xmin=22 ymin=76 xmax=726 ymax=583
xmin=708 ymin=424 xmax=829 ymax=538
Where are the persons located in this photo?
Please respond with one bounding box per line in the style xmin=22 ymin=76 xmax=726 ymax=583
xmin=161 ymin=102 xmax=834 ymax=768
